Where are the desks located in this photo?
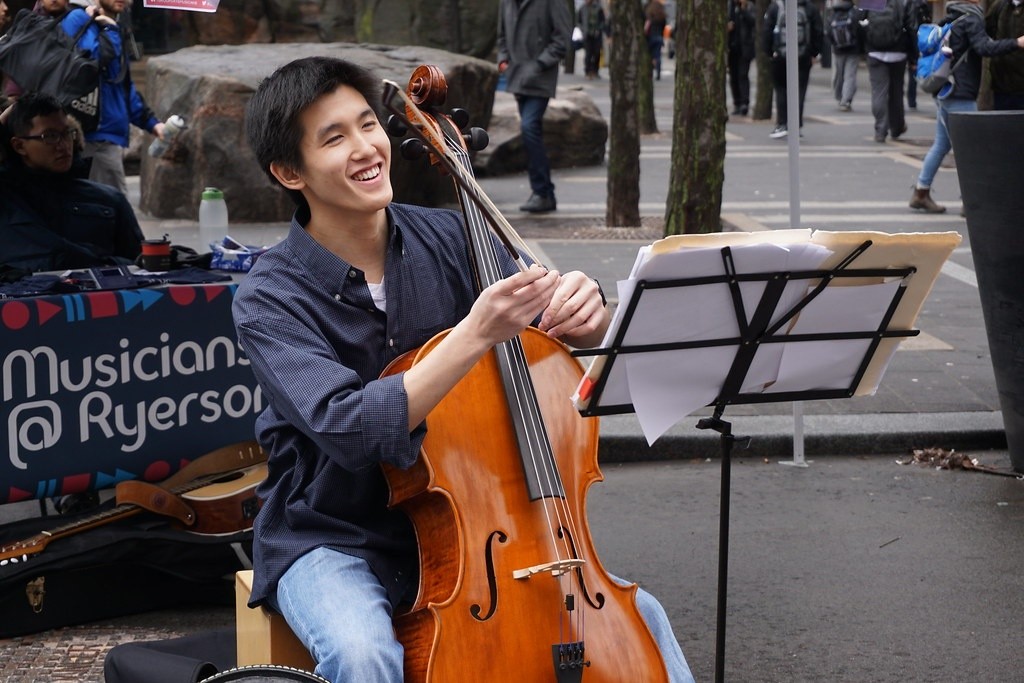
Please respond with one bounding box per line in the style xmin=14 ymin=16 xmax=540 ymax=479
xmin=1 ymin=270 xmax=269 ymax=504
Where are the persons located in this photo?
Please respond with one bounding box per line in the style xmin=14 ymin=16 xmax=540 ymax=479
xmin=231 ymin=57 xmax=696 ymax=683
xmin=580 ymin=0 xmax=1024 ymax=214
xmin=0 ymin=0 xmax=178 ymax=515
xmin=494 ymin=0 xmax=573 ymax=215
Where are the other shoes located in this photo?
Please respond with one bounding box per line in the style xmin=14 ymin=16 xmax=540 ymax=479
xmin=519 ymin=190 xmax=556 ymax=214
xmin=875 ymin=132 xmax=885 ymax=143
xmin=769 ymin=125 xmax=788 ymax=138
xmin=732 ymin=106 xmax=749 ymax=116
xmin=891 ymin=124 xmax=907 ymax=138
xmin=840 ymin=103 xmax=853 ymax=111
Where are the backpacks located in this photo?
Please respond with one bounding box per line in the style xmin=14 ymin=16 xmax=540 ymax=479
xmin=866 ymin=0 xmax=906 ymax=52
xmin=774 ymin=0 xmax=810 ymax=57
xmin=916 ymin=13 xmax=977 ymax=100
xmin=831 ymin=9 xmax=855 ymax=48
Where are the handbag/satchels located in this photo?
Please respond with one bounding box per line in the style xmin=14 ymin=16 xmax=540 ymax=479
xmin=0 ymin=6 xmax=112 ymax=141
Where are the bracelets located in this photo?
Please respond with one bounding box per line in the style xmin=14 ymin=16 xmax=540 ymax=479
xmin=590 ymin=277 xmax=608 ymax=308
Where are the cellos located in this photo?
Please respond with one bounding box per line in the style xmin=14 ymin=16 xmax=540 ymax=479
xmin=376 ymin=64 xmax=670 ymax=683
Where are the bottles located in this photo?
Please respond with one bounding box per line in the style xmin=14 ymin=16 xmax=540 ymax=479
xmin=147 ymin=114 xmax=185 ymax=158
xmin=200 ymin=187 xmax=228 ymax=243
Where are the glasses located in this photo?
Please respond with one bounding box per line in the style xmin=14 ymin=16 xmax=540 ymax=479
xmin=20 ymin=127 xmax=79 ymax=143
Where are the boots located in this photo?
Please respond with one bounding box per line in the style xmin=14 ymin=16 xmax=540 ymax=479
xmin=909 ymin=187 xmax=946 ymax=213
xmin=959 ymin=201 xmax=967 ymax=217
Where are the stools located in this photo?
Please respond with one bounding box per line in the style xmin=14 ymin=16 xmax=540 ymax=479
xmin=236 ymin=569 xmax=317 ymax=674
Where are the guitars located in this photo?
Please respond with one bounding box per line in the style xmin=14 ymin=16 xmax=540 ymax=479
xmin=0 ymin=455 xmax=270 ymax=568
